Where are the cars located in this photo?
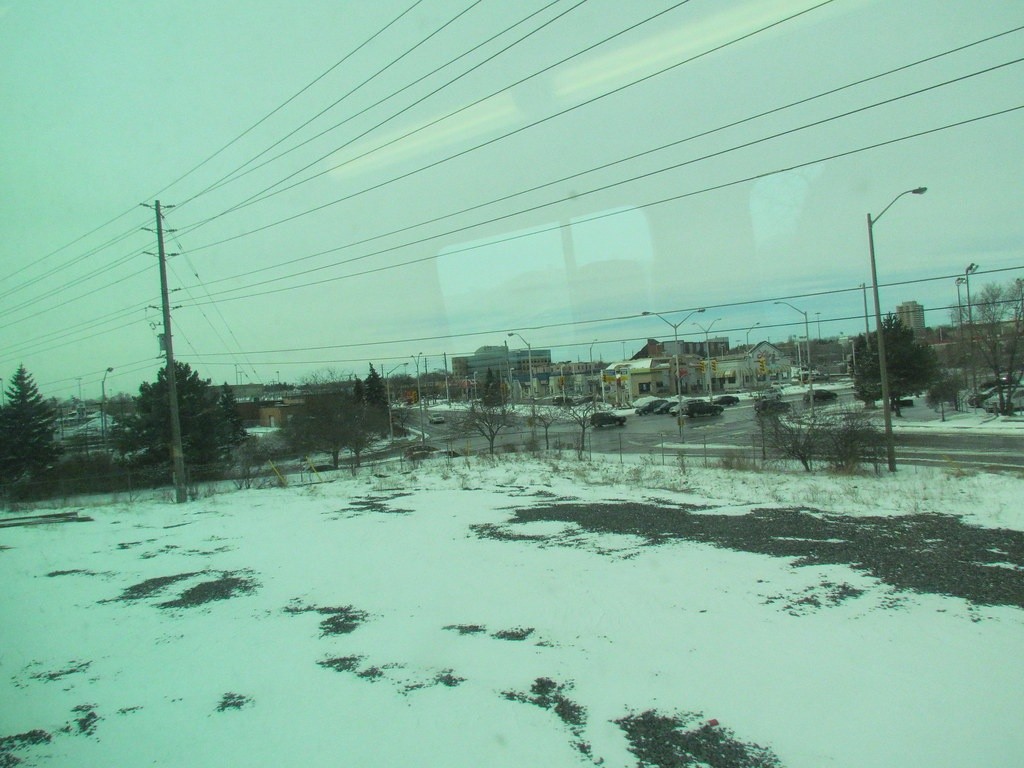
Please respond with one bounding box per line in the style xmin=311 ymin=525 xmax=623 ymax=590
xmin=754 ymin=387 xmax=782 ymax=400
xmin=669 ymin=398 xmax=705 ymax=416
xmin=799 ymin=366 xmax=821 ymax=376
xmin=708 ymin=396 xmax=740 ymax=406
xmin=967 ymin=377 xmax=1020 ymax=408
xmin=635 ymin=400 xmax=668 ymax=416
xmin=770 ymin=382 xmax=781 ymax=390
xmin=804 ymin=389 xmax=838 ymax=402
xmin=976 ymin=384 xmax=1024 ymax=408
xmin=572 ymin=396 xmax=593 ymax=406
xmin=780 ymin=381 xmax=792 ymax=389
xmin=753 ymin=400 xmax=791 ymax=414
xmin=653 ymin=401 xmax=679 ymax=415
xmin=997 ymin=387 xmax=1024 ymax=412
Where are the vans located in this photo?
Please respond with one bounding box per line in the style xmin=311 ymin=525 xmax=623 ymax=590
xmin=552 ymin=396 xmax=575 ymax=405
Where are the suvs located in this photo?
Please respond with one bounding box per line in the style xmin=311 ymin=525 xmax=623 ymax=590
xmin=590 ymin=412 xmax=627 ymax=427
xmin=683 ymin=402 xmax=724 ymax=418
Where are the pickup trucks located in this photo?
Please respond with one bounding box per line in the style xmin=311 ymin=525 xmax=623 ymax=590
xmin=427 ymin=413 xmax=445 ymax=424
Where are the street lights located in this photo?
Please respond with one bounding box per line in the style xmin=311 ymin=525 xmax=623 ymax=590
xmin=589 ymin=339 xmax=598 ymax=414
xmin=849 ymin=340 xmax=856 ymax=366
xmin=465 ymin=376 xmax=469 ymax=402
xmin=234 ymin=364 xmax=238 ymax=385
xmin=642 ymin=308 xmax=706 ymax=444
xmin=814 ymin=312 xmax=824 ymax=368
xmin=473 ymin=372 xmax=478 ymax=401
xmin=859 ymin=283 xmax=871 ymax=355
xmin=276 ymin=371 xmax=280 ymax=385
xmin=955 ymin=278 xmax=969 ymax=394
xmin=101 ymin=368 xmax=114 ymax=454
xmin=746 ymin=322 xmax=760 ymax=397
xmin=866 ymin=188 xmax=929 ymax=472
xmin=387 ymin=363 xmax=409 ymax=446
xmin=445 ymin=376 xmax=450 ymax=402
xmin=509 ymin=367 xmax=514 ymax=399
xmin=560 ymin=363 xmax=566 ymax=405
xmin=508 ymin=332 xmax=538 ymax=441
xmin=774 ymin=301 xmax=817 ymax=419
xmin=691 ymin=318 xmax=721 ymax=403
xmin=411 ymin=352 xmax=425 ymax=446
xmin=966 ymin=263 xmax=980 ymax=396
xmin=793 ymin=333 xmax=805 ymax=387
